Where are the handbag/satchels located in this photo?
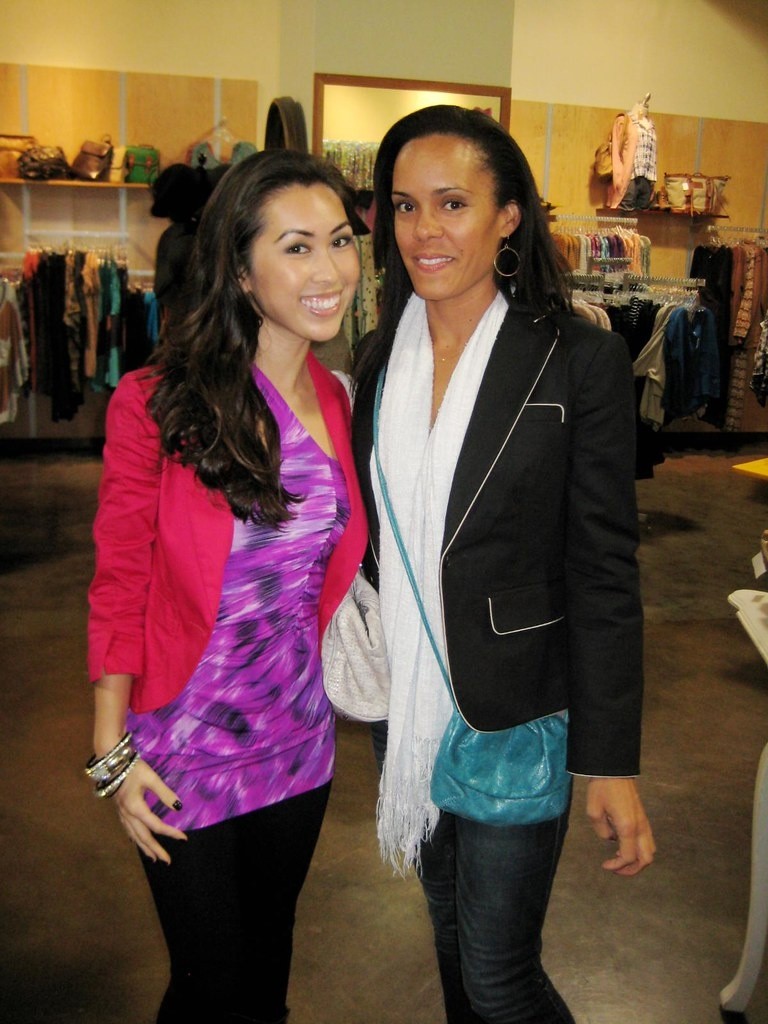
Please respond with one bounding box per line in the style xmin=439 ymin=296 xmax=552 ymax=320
xmin=319 ymin=369 xmax=392 ymax=723
xmin=593 ymin=112 xmax=629 ymax=183
xmin=664 ymin=171 xmax=730 ymax=215
xmin=11 ymin=131 xmax=257 ymax=184
xmin=429 ymin=708 xmax=570 ymax=827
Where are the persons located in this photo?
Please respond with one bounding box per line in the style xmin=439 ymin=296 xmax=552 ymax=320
xmin=85 ymin=147 xmax=370 ymax=1024
xmin=605 ymin=103 xmax=657 ymax=213
xmin=150 ymin=164 xmax=208 ymax=309
xmin=349 ymin=105 xmax=657 ymax=1024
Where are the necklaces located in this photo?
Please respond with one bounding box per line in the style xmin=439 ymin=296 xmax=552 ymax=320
xmin=441 ymin=349 xmax=463 ymax=363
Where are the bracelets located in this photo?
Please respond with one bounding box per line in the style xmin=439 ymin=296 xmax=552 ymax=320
xmin=84 ymin=731 xmax=139 ymax=799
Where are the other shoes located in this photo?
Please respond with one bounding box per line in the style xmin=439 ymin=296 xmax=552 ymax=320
xmin=649 ymin=190 xmax=671 ymax=212
